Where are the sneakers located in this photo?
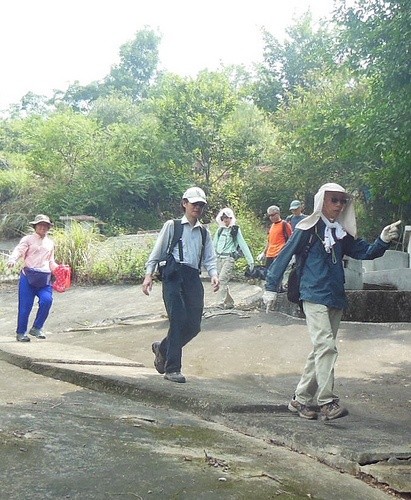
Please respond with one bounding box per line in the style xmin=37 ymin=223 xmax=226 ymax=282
xmin=164 ymin=371 xmax=185 ymax=383
xmin=320 ymin=400 xmax=348 ymax=420
xmin=288 ymin=394 xmax=317 ymax=418
xmin=152 ymin=342 xmax=166 ymax=374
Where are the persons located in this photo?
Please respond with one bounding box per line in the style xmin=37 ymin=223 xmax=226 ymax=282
xmin=281 ymin=200 xmax=309 ymax=290
xmin=257 ymin=206 xmax=293 ymax=293
xmin=261 ymin=182 xmax=402 ymax=420
xmin=212 ymin=207 xmax=254 ymax=309
xmin=6 ymin=215 xmax=69 ymax=343
xmin=140 ymin=187 xmax=221 ymax=382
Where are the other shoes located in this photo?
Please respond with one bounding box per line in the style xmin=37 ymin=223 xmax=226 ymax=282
xmin=29 ymin=326 xmax=46 ymax=339
xmin=16 ymin=334 xmax=30 ymax=342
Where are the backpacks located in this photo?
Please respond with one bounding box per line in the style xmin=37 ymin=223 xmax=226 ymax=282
xmin=155 ymin=219 xmax=207 ymax=281
xmin=232 ymin=226 xmax=245 ymax=258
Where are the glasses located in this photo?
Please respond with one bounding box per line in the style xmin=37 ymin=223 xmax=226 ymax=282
xmin=192 ymin=202 xmax=205 ymax=207
xmin=330 ymin=197 xmax=347 ymax=204
xmin=268 ymin=213 xmax=277 ymax=217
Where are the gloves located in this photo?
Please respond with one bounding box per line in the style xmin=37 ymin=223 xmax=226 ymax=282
xmin=257 ymin=252 xmax=265 ymax=261
xmin=380 ymin=220 xmax=402 ymax=243
xmin=289 ymin=254 xmax=296 ymax=265
xmin=262 ymin=291 xmax=277 ymax=314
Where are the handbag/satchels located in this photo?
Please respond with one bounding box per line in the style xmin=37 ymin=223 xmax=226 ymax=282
xmin=287 ymin=223 xmax=315 ymax=303
xmin=52 ymin=265 xmax=67 ymax=292
xmin=65 ymin=268 xmax=71 ymax=288
xmin=244 ymin=260 xmax=268 ymax=280
xmin=24 ymin=267 xmax=51 ymax=287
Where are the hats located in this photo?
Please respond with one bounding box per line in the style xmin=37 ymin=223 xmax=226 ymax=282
xmin=182 ymin=187 xmax=208 ymax=203
xmin=289 ymin=200 xmax=301 ymax=210
xmin=325 ymin=190 xmax=353 ymax=198
xmin=29 ymin=214 xmax=54 ymax=226
